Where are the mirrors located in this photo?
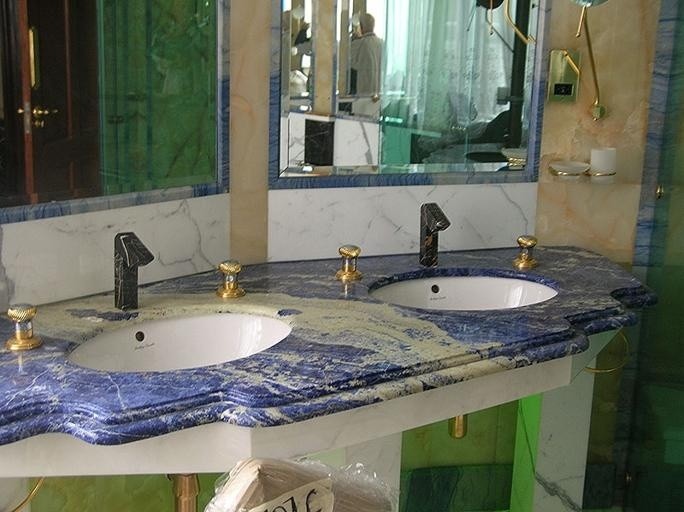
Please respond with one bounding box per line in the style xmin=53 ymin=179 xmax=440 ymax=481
xmin=0 ymin=0 xmax=232 ymax=224
xmin=268 ymin=2 xmax=550 ymax=191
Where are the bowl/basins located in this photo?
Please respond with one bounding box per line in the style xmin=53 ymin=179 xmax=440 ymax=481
xmin=549 ymin=159 xmax=590 ymax=181
xmin=501 ymin=148 xmax=529 ymax=167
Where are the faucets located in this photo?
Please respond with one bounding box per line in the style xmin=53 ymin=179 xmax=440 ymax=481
xmin=114 ymin=232 xmax=154 ymax=310
xmin=419 ymin=201 xmax=450 ymax=268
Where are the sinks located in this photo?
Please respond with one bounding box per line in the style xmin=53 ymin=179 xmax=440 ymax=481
xmin=368 ymin=269 xmax=558 ymax=311
xmin=63 ymin=312 xmax=293 ymax=373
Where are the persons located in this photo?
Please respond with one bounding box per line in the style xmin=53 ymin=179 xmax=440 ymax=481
xmin=349 ymin=13 xmax=388 ymax=95
xmin=293 ymin=15 xmax=312 ymax=93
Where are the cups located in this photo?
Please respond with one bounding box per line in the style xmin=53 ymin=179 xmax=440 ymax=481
xmin=589 ymin=146 xmax=619 ymax=187
